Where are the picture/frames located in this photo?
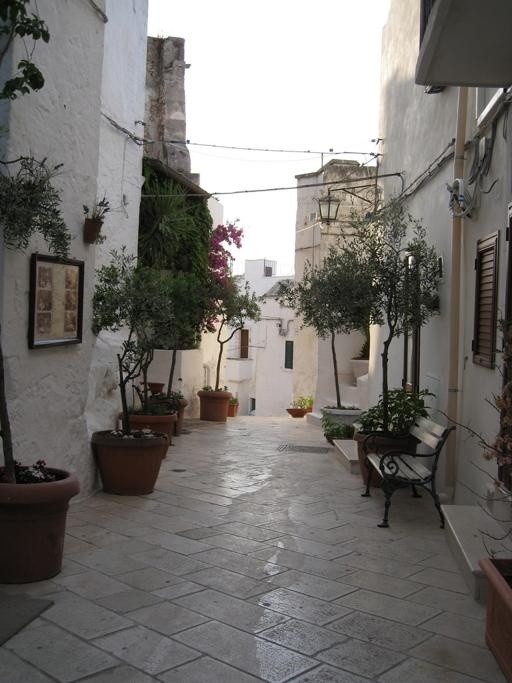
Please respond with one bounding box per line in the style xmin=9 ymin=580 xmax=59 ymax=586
xmin=25 ymin=252 xmax=86 ymax=350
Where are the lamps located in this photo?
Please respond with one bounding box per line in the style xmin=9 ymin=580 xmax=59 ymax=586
xmin=314 ymin=181 xmax=385 ymax=229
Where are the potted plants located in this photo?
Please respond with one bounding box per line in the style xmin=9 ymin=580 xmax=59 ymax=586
xmin=0 ymin=153 xmax=83 ymax=587
xmin=436 ymin=214 xmax=512 ymax=683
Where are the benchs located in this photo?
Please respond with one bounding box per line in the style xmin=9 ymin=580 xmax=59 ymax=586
xmin=361 ymin=416 xmax=452 ymax=529
